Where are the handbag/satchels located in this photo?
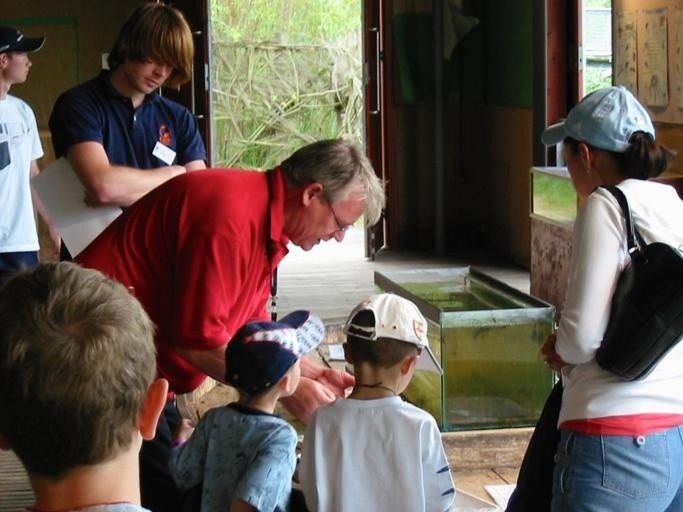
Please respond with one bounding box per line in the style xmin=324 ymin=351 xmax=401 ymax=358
xmin=590 ymin=182 xmax=683 ymax=382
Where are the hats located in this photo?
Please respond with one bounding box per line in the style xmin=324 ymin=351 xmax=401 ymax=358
xmin=0 ymin=27 xmax=44 ymax=54
xmin=225 ymin=310 xmax=325 ymax=391
xmin=344 ymin=292 xmax=443 ymax=375
xmin=541 ymin=85 xmax=656 ymax=153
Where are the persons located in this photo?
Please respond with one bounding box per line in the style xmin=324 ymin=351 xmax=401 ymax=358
xmin=1 ymin=261 xmax=169 ymax=512
xmin=70 ymin=139 xmax=387 ymax=425
xmin=541 ymin=86 xmax=683 ymax=512
xmin=299 ymin=293 xmax=456 ymax=512
xmin=0 ymin=25 xmax=45 ymax=292
xmin=49 ymin=0 xmax=208 ymax=263
xmin=170 ymin=309 xmax=326 ymax=511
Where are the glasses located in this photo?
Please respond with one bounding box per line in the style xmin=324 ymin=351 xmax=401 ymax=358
xmin=324 ymin=194 xmax=352 ymax=231
